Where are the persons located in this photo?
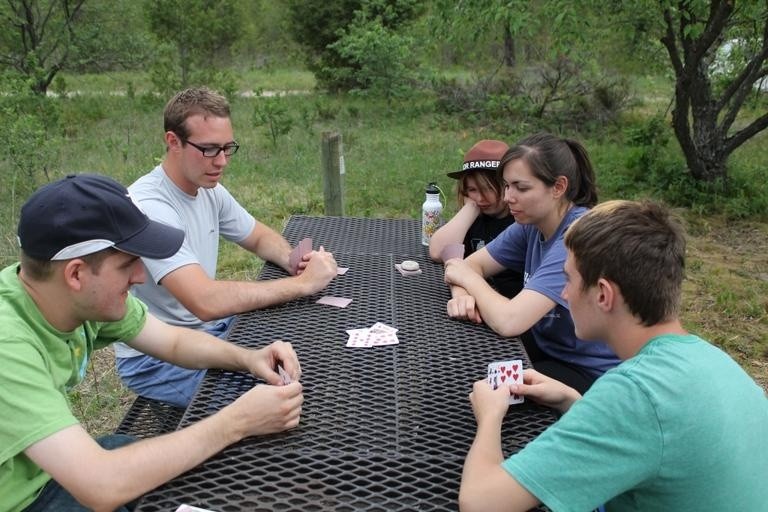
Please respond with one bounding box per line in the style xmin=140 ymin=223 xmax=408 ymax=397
xmin=455 ymin=191 xmax=766 ymax=512
xmin=427 ymin=138 xmax=527 ymax=294
xmin=0 ymin=171 xmax=308 ymax=510
xmin=436 ymin=133 xmax=625 ymax=399
xmin=111 ymin=87 xmax=342 ymax=408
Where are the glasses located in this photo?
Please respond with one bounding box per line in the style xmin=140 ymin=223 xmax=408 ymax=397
xmin=186 ymin=139 xmax=239 ymax=156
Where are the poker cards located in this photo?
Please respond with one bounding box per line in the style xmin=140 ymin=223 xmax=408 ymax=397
xmin=488 ymin=359 xmax=525 ymax=406
xmin=438 ymin=242 xmax=466 ymax=263
xmin=277 ymin=365 xmax=292 ymax=386
xmin=317 ymin=296 xmax=351 ymax=307
xmin=346 ymin=322 xmax=399 ymax=348
xmin=289 ymin=239 xmax=313 ymax=277
xmin=337 ymin=267 xmax=348 ymax=275
xmin=176 ymin=503 xmax=213 ymax=511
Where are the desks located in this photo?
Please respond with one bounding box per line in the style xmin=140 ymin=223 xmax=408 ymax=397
xmin=133 ymin=214 xmax=597 ymax=511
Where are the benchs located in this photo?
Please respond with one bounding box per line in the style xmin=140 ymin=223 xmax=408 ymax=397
xmin=115 ymin=396 xmax=186 ymax=439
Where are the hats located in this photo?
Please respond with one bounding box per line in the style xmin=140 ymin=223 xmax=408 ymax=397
xmin=16 ymin=175 xmax=185 ymax=261
xmin=447 ymin=139 xmax=510 ymax=179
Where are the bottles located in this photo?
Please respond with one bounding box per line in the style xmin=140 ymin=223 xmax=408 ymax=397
xmin=420 ymin=185 xmax=444 ymax=246
